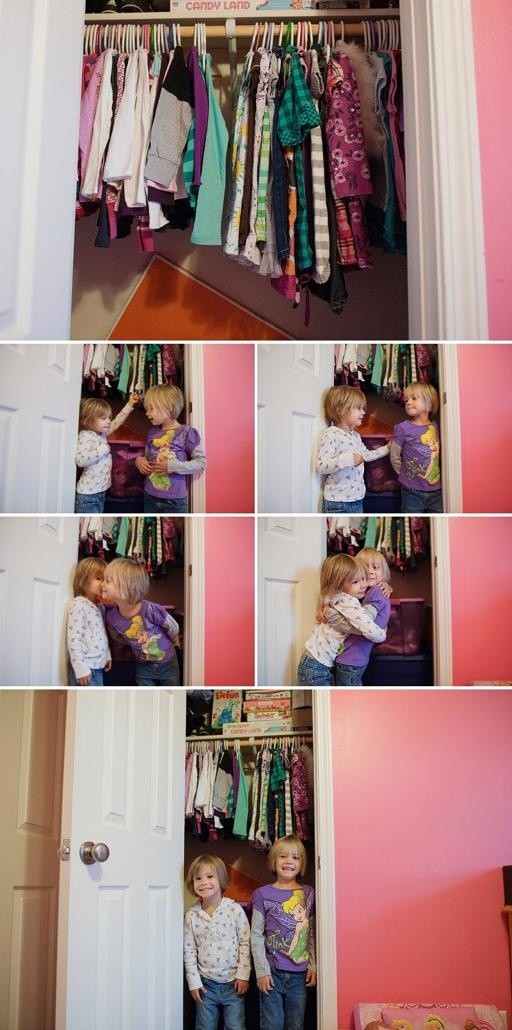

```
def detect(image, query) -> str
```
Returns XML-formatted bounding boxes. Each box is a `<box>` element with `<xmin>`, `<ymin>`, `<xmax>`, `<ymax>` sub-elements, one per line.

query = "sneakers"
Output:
<box><xmin>190</xmin><ymin>725</ymin><xmax>210</xmax><ymax>735</ymax></box>
<box><xmin>104</xmin><ymin>0</ymin><xmax>145</xmax><ymax>11</ymax></box>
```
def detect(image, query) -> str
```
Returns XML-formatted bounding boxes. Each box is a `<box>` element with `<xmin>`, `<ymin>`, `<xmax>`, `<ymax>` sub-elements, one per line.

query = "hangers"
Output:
<box><xmin>84</xmin><ymin>19</ymin><xmax>403</xmax><ymax>91</ymax></box>
<box><xmin>186</xmin><ymin>737</ymin><xmax>311</xmax><ymax>757</ymax></box>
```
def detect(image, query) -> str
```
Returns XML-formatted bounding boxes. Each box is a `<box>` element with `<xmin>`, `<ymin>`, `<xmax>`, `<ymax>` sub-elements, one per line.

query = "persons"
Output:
<box><xmin>297</xmin><ymin>553</ymin><xmax>392</xmax><ymax>686</ymax></box>
<box><xmin>250</xmin><ymin>833</ymin><xmax>317</xmax><ymax>1029</ymax></box>
<box><xmin>315</xmin><ymin>385</ymin><xmax>392</xmax><ymax>513</ymax></box>
<box><xmin>391</xmin><ymin>380</ymin><xmax>444</xmax><ymax>513</ymax></box>
<box><xmin>101</xmin><ymin>558</ymin><xmax>182</xmax><ymax>686</ymax></box>
<box><xmin>183</xmin><ymin>853</ymin><xmax>252</xmax><ymax>1030</ymax></box>
<box><xmin>134</xmin><ymin>383</ymin><xmax>206</xmax><ymax>513</ymax></box>
<box><xmin>67</xmin><ymin>557</ymin><xmax>113</xmax><ymax>686</ymax></box>
<box><xmin>316</xmin><ymin>548</ymin><xmax>391</xmax><ymax>686</ymax></box>
<box><xmin>75</xmin><ymin>391</ymin><xmax>139</xmax><ymax>513</ymax></box>
